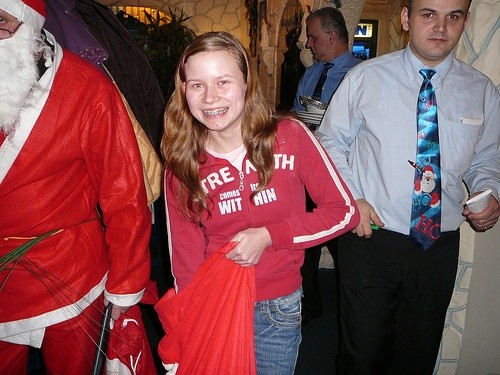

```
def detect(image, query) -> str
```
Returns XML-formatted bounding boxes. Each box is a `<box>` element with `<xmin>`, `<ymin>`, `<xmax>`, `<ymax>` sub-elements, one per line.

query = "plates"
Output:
<box><xmin>296</xmin><ymin>110</ymin><xmax>325</xmax><ymax>125</ymax></box>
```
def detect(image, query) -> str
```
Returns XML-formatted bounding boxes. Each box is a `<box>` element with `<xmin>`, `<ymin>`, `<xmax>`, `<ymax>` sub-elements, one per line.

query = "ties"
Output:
<box><xmin>409</xmin><ymin>68</ymin><xmax>442</xmax><ymax>253</ymax></box>
<box><xmin>311</xmin><ymin>63</ymin><xmax>335</xmax><ymax>100</ymax></box>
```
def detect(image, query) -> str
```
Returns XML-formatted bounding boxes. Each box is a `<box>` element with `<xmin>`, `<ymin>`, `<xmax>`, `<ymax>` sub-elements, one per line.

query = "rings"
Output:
<box><xmin>484</xmin><ymin>226</ymin><xmax>488</xmax><ymax>230</ymax></box>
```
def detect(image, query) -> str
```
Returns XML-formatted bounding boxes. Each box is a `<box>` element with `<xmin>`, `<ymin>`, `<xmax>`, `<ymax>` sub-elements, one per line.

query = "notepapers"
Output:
<box><xmin>465</xmin><ymin>187</ymin><xmax>494</xmax><ymax>214</ymax></box>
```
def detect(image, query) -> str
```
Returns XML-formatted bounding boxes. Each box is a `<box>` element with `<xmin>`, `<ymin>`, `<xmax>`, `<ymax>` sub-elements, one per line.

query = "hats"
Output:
<box><xmin>0</xmin><ymin>0</ymin><xmax>47</xmax><ymax>34</ymax></box>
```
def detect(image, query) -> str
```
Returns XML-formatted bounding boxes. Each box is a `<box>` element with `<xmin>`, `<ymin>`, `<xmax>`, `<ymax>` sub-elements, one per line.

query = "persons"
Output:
<box><xmin>312</xmin><ymin>0</ymin><xmax>499</xmax><ymax>375</ymax></box>
<box><xmin>159</xmin><ymin>30</ymin><xmax>360</xmax><ymax>375</ymax></box>
<box><xmin>288</xmin><ymin>6</ymin><xmax>365</xmax><ymax>329</ymax></box>
<box><xmin>0</xmin><ymin>0</ymin><xmax>151</xmax><ymax>375</ymax></box>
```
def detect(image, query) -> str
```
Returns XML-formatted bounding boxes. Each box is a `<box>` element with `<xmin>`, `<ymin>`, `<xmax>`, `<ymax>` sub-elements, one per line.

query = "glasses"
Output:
<box><xmin>0</xmin><ymin>22</ymin><xmax>23</xmax><ymax>40</ymax></box>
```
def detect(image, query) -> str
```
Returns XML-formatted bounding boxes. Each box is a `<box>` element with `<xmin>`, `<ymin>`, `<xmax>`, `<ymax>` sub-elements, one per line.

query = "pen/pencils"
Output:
<box><xmin>408</xmin><ymin>160</ymin><xmax>425</xmax><ymax>173</ymax></box>
<box><xmin>371</xmin><ymin>224</ymin><xmax>380</xmax><ymax>230</ymax></box>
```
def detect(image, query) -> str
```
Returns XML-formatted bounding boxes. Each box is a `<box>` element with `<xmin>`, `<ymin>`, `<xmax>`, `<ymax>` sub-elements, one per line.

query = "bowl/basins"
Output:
<box><xmin>299</xmin><ymin>95</ymin><xmax>327</xmax><ymax>110</ymax></box>
